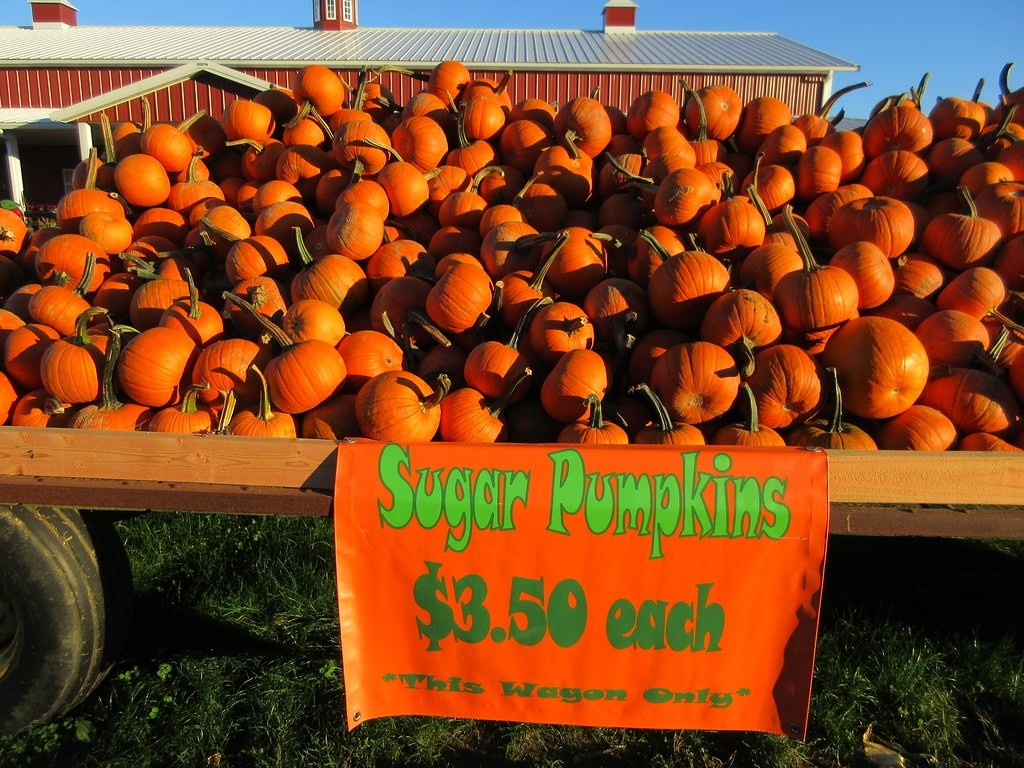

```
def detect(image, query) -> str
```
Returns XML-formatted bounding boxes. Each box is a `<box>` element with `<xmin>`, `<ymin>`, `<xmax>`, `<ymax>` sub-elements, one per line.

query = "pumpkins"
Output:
<box><xmin>0</xmin><ymin>61</ymin><xmax>1024</xmax><ymax>451</ymax></box>
